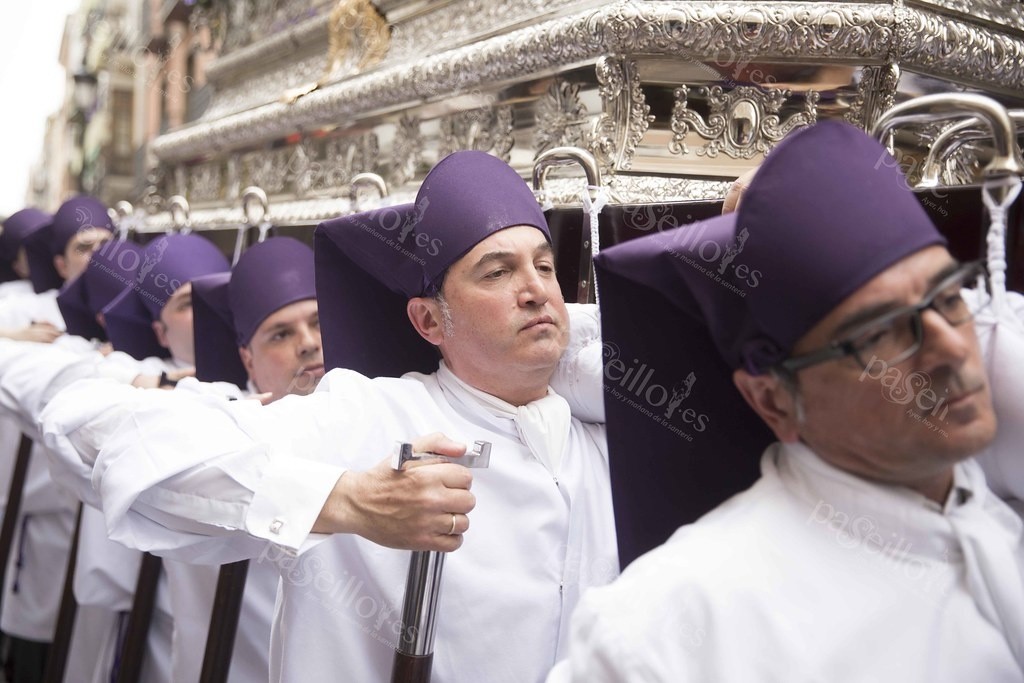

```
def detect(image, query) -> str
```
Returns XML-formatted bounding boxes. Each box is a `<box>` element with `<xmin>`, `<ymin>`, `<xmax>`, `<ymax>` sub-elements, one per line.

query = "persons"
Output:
<box><xmin>103</xmin><ymin>148</ymin><xmax>760</xmax><ymax>681</ymax></box>
<box><xmin>546</xmin><ymin>115</ymin><xmax>1024</xmax><ymax>683</ymax></box>
<box><xmin>0</xmin><ymin>194</ymin><xmax>325</xmax><ymax>683</ymax></box>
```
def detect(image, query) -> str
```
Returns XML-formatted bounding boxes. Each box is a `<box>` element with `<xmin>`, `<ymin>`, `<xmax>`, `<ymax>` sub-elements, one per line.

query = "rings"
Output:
<box><xmin>448</xmin><ymin>512</ymin><xmax>458</xmax><ymax>537</ymax></box>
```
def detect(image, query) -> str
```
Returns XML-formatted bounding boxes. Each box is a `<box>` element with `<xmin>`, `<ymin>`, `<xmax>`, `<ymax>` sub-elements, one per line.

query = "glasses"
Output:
<box><xmin>779</xmin><ymin>258</ymin><xmax>992</xmax><ymax>377</ymax></box>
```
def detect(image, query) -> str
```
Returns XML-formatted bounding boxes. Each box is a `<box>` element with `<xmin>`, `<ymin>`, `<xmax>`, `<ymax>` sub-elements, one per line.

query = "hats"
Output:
<box><xmin>1</xmin><ymin>150</ymin><xmax>556</xmax><ymax>392</ymax></box>
<box><xmin>591</xmin><ymin>116</ymin><xmax>950</xmax><ymax>576</ymax></box>
